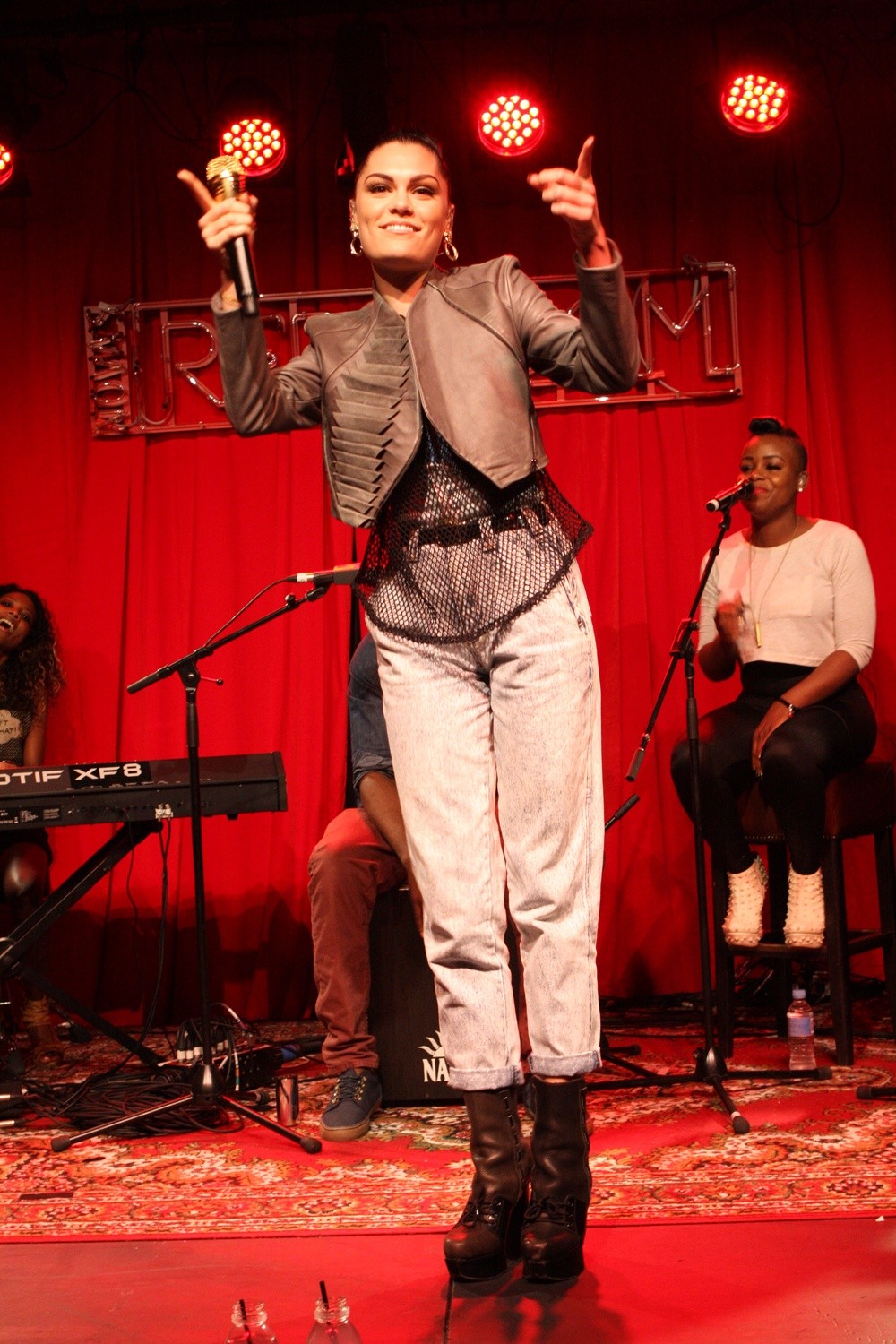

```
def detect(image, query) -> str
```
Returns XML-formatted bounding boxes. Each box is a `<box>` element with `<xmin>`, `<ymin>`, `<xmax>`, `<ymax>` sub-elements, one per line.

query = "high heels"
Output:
<box><xmin>783</xmin><ymin>863</ymin><xmax>826</xmax><ymax>948</ymax></box>
<box><xmin>721</xmin><ymin>853</ymin><xmax>769</xmax><ymax>947</ymax></box>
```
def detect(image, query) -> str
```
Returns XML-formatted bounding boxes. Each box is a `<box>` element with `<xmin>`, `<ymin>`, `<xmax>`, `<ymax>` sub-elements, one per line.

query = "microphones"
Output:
<box><xmin>204</xmin><ymin>155</ymin><xmax>262</xmax><ymax>318</ymax></box>
<box><xmin>286</xmin><ymin>559</ymin><xmax>386</xmax><ymax>588</ymax></box>
<box><xmin>705</xmin><ymin>477</ymin><xmax>755</xmax><ymax>513</ymax></box>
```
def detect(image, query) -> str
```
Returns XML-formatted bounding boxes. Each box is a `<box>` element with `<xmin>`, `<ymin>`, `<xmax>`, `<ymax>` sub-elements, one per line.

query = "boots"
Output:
<box><xmin>445</xmin><ymin>1089</ymin><xmax>529</xmax><ymax>1285</ymax></box>
<box><xmin>521</xmin><ymin>1074</ymin><xmax>593</xmax><ymax>1283</ymax></box>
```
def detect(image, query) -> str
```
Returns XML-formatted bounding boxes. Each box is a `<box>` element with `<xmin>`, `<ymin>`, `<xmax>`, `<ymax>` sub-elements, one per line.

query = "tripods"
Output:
<box><xmin>589</xmin><ymin>518</ymin><xmax>834</xmax><ymax>1138</ymax></box>
<box><xmin>50</xmin><ymin>587</ymin><xmax>326</xmax><ymax>1157</ymax></box>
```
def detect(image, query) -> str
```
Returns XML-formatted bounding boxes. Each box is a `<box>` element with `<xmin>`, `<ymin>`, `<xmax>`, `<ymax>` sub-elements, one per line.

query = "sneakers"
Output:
<box><xmin>319</xmin><ymin>1067</ymin><xmax>384</xmax><ymax>1140</ymax></box>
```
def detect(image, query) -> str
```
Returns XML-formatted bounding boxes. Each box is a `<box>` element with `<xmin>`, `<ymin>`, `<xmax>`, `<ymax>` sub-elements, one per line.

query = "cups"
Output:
<box><xmin>276</xmin><ymin>1075</ymin><xmax>299</xmax><ymax>1126</ymax></box>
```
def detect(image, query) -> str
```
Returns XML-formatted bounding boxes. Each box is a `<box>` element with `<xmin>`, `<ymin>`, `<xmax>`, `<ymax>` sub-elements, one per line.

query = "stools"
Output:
<box><xmin>714</xmin><ymin>760</ymin><xmax>896</xmax><ymax>1067</ymax></box>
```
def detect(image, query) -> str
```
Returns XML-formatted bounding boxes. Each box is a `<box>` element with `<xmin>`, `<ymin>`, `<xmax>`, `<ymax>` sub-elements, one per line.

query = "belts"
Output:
<box><xmin>402</xmin><ymin>509</ymin><xmax>549</xmax><ymax>547</ymax></box>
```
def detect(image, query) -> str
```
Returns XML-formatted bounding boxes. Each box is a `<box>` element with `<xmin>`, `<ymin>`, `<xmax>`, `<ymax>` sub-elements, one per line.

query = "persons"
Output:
<box><xmin>668</xmin><ymin>417</ymin><xmax>877</xmax><ymax>949</ymax></box>
<box><xmin>307</xmin><ymin>640</ymin><xmax>412</xmax><ymax>1140</ymax></box>
<box><xmin>169</xmin><ymin>126</ymin><xmax>640</xmax><ymax>1294</ymax></box>
<box><xmin>0</xmin><ymin>579</ymin><xmax>66</xmax><ymax>971</ymax></box>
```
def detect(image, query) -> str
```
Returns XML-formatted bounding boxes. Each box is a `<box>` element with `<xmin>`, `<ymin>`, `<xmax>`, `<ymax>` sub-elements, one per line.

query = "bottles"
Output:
<box><xmin>226</xmin><ymin>1298</ymin><xmax>278</xmax><ymax>1344</ymax></box>
<box><xmin>306</xmin><ymin>1298</ymin><xmax>363</xmax><ymax>1344</ymax></box>
<box><xmin>785</xmin><ymin>989</ymin><xmax>818</xmax><ymax>1070</ymax></box>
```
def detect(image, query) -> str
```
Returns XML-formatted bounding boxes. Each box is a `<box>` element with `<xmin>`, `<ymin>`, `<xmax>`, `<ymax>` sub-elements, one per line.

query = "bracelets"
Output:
<box><xmin>775</xmin><ymin>695</ymin><xmax>801</xmax><ymax>716</ymax></box>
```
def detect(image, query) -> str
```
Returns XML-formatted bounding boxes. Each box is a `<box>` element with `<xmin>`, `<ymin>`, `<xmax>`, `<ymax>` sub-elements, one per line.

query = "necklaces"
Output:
<box><xmin>748</xmin><ymin>510</ymin><xmax>802</xmax><ymax>650</ymax></box>
